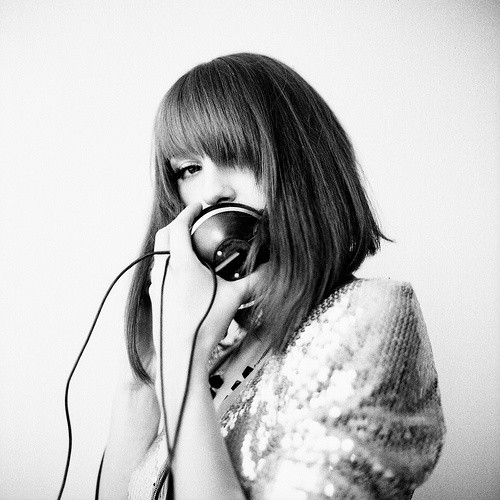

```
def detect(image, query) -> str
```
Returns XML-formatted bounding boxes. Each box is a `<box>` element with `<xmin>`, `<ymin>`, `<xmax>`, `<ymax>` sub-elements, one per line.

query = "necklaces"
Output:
<box><xmin>150</xmin><ymin>314</ymin><xmax>274</xmax><ymax>500</ymax></box>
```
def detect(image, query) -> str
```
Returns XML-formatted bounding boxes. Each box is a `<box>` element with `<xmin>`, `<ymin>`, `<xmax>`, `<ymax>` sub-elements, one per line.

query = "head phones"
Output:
<box><xmin>187</xmin><ymin>203</ymin><xmax>267</xmax><ymax>310</ymax></box>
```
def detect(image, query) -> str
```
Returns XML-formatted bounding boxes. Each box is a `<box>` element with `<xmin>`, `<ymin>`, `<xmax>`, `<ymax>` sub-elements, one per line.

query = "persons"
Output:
<box><xmin>94</xmin><ymin>52</ymin><xmax>448</xmax><ymax>500</ymax></box>
